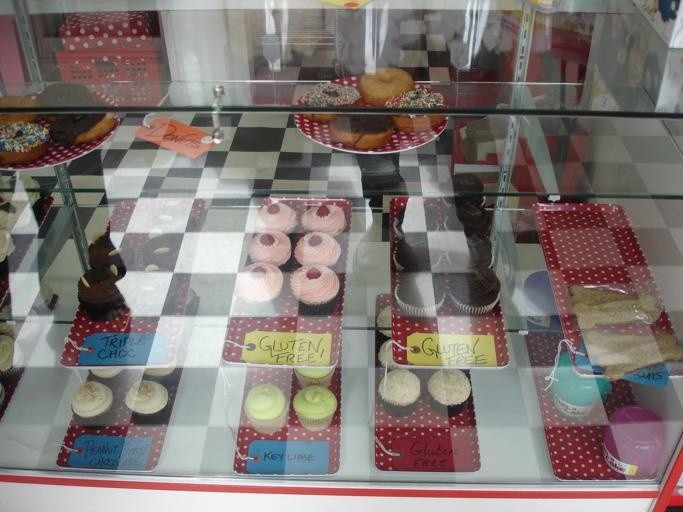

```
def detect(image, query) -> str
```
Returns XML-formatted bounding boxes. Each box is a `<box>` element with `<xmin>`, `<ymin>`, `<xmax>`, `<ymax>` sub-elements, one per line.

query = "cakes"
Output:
<box><xmin>0</xmin><ymin>173</ymin><xmax>54</xmax><ymax>409</ymax></box>
<box><xmin>1</xmin><ymin>174</ymin><xmax>50</xmax><ymax>401</ymax></box>
<box><xmin>69</xmin><ymin>170</ymin><xmax>502</xmax><ymax>434</ymax></box>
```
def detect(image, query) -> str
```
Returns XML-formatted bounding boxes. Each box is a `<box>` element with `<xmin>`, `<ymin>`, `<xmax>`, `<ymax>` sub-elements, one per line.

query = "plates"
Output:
<box><xmin>58</xmin><ymin>197</ymin><xmax>205</xmax><ymax>369</ymax></box>
<box><xmin>373</xmin><ymin>291</ymin><xmax>482</xmax><ymax>474</ymax></box>
<box><xmin>0</xmin><ymin>288</ymin><xmax>202</xmax><ymax>475</ymax></box>
<box><xmin>292</xmin><ymin>74</ymin><xmax>450</xmax><ymax>157</ymax></box>
<box><xmin>231</xmin><ymin>332</ymin><xmax>343</xmax><ymax>477</ymax></box>
<box><xmin>388</xmin><ymin>195</ymin><xmax>510</xmax><ymax>370</ymax></box>
<box><xmin>0</xmin><ymin>89</ymin><xmax>120</xmax><ymax>171</ymax></box>
<box><xmin>531</xmin><ymin>202</ymin><xmax>683</xmax><ymax>378</ymax></box>
<box><xmin>524</xmin><ymin>331</ymin><xmax>660</xmax><ymax>483</ymax></box>
<box><xmin>221</xmin><ymin>196</ymin><xmax>351</xmax><ymax>369</ymax></box>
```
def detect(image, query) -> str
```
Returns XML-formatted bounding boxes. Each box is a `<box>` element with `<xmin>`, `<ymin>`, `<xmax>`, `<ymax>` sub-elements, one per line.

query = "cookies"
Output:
<box><xmin>570</xmin><ymin>285</ymin><xmax>682</xmax><ymax>382</ymax></box>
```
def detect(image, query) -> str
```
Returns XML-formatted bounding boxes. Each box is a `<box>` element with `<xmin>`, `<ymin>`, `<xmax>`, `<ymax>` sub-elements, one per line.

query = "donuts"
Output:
<box><xmin>1</xmin><ymin>95</ymin><xmax>40</xmax><ymax>123</ymax></box>
<box><xmin>357</xmin><ymin>66</ymin><xmax>416</xmax><ymax>108</ymax></box>
<box><xmin>328</xmin><ymin>103</ymin><xmax>396</xmax><ymax>148</ymax></box>
<box><xmin>304</xmin><ymin>83</ymin><xmax>363</xmax><ymax>126</ymax></box>
<box><xmin>49</xmin><ymin>97</ymin><xmax>114</xmax><ymax>145</ymax></box>
<box><xmin>0</xmin><ymin>83</ymin><xmax>114</xmax><ymax>167</ymax></box>
<box><xmin>42</xmin><ymin>83</ymin><xmax>92</xmax><ymax>124</ymax></box>
<box><xmin>0</xmin><ymin>120</ymin><xmax>50</xmax><ymax>165</ymax></box>
<box><xmin>382</xmin><ymin>86</ymin><xmax>445</xmax><ymax>134</ymax></box>
<box><xmin>304</xmin><ymin>64</ymin><xmax>447</xmax><ymax>149</ymax></box>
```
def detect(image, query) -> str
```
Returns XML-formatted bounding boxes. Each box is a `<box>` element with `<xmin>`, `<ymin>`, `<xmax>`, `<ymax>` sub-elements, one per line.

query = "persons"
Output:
<box><xmin>322</xmin><ymin>3</ymin><xmax>521</xmax><ymax>301</ymax></box>
<box><xmin>0</xmin><ymin>0</ymin><xmax>127</xmax><ymax>370</ymax></box>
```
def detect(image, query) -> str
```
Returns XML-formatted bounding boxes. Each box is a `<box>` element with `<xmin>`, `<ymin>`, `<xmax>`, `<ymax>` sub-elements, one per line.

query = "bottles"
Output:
<box><xmin>550</xmin><ymin>351</ymin><xmax>614</xmax><ymax>422</ymax></box>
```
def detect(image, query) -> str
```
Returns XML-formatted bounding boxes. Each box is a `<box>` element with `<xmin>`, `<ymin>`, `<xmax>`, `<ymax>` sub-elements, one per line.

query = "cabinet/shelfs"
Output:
<box><xmin>0</xmin><ymin>0</ymin><xmax>683</xmax><ymax>512</ymax></box>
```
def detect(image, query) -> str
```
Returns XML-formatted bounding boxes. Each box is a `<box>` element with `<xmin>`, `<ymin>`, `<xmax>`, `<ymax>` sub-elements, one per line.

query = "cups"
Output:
<box><xmin>601</xmin><ymin>404</ymin><xmax>667</xmax><ymax>481</ymax></box>
